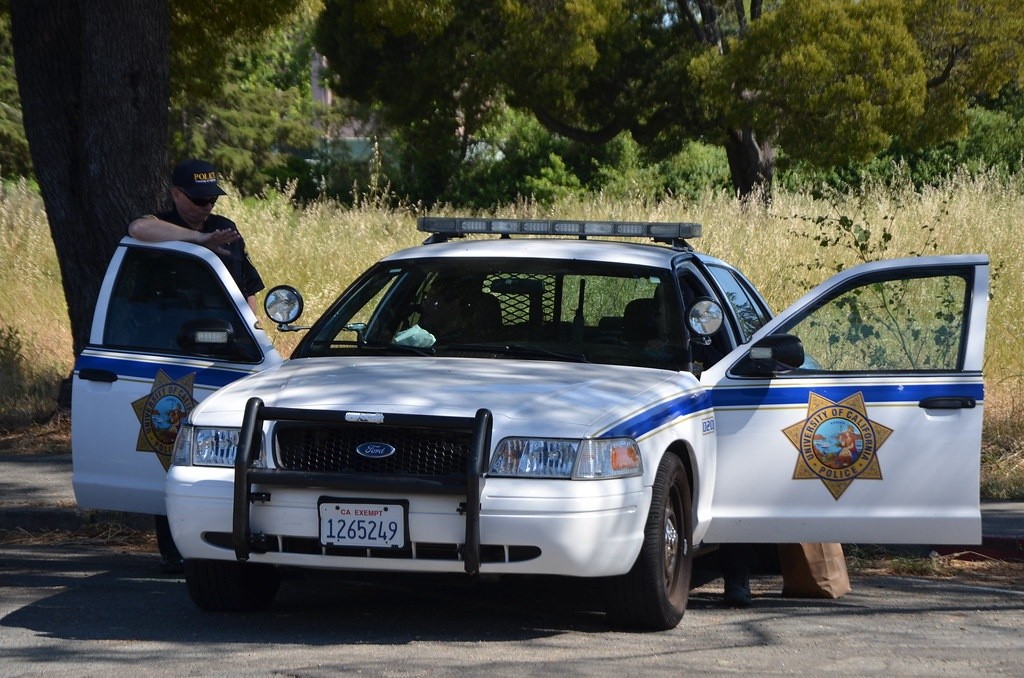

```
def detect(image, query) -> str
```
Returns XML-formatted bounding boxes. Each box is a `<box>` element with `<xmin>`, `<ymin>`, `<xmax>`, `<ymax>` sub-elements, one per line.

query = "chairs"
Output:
<box><xmin>621</xmin><ymin>298</ymin><xmax>657</xmax><ymax>343</ymax></box>
<box><xmin>418</xmin><ymin>273</ymin><xmax>502</xmax><ymax>346</ymax></box>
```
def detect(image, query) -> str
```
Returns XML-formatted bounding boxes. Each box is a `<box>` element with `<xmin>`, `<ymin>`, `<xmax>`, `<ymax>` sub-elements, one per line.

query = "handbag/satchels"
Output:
<box><xmin>776</xmin><ymin>542</ymin><xmax>853</xmax><ymax>600</ymax></box>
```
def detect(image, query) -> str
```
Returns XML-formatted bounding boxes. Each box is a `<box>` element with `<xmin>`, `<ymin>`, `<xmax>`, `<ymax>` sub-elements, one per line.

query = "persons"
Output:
<box><xmin>624</xmin><ymin>281</ymin><xmax>753</xmax><ymax>607</ymax></box>
<box><xmin>127</xmin><ymin>159</ymin><xmax>264</xmax><ymax>568</ymax></box>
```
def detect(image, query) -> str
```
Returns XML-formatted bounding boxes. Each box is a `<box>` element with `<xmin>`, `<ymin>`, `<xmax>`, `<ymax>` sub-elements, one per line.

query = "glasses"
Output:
<box><xmin>177</xmin><ymin>187</ymin><xmax>219</xmax><ymax>207</ymax></box>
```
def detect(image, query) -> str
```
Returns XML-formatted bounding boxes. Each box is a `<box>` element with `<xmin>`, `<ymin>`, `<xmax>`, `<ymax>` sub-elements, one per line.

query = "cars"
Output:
<box><xmin>68</xmin><ymin>214</ymin><xmax>992</xmax><ymax>632</ymax></box>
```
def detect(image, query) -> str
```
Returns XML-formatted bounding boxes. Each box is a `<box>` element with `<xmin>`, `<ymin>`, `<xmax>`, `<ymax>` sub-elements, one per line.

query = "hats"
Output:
<box><xmin>173</xmin><ymin>160</ymin><xmax>228</xmax><ymax>198</ymax></box>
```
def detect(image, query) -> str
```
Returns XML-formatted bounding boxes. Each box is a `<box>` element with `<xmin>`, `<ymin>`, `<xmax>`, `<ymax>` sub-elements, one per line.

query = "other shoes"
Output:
<box><xmin>163</xmin><ymin>553</ymin><xmax>183</xmax><ymax>574</ymax></box>
<box><xmin>724</xmin><ymin>582</ymin><xmax>754</xmax><ymax>607</ymax></box>
